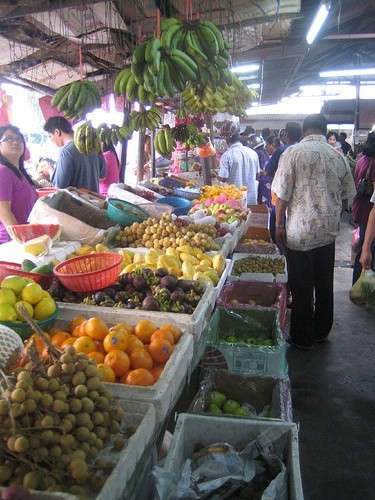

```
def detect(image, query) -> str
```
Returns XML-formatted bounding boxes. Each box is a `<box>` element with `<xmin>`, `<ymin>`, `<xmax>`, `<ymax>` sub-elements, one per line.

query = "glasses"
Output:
<box><xmin>0</xmin><ymin>136</ymin><xmax>23</xmax><ymax>143</ymax></box>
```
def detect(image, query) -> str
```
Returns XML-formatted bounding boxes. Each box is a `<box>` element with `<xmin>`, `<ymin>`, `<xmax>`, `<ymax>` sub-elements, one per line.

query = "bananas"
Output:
<box><xmin>171</xmin><ymin>123</ymin><xmax>198</xmax><ymax>148</ymax></box>
<box><xmin>110</xmin><ymin>123</ymin><xmax>131</xmax><ymax>145</ymax></box>
<box><xmin>184</xmin><ymin>131</ymin><xmax>210</xmax><ymax>150</ymax></box>
<box><xmin>114</xmin><ymin>18</ymin><xmax>260</xmax><ymax>118</ymax></box>
<box><xmin>154</xmin><ymin>124</ymin><xmax>176</xmax><ymax>159</ymax></box>
<box><xmin>51</xmin><ymin>81</ymin><xmax>103</xmax><ymax>122</ymax></box>
<box><xmin>129</xmin><ymin>107</ymin><xmax>167</xmax><ymax>132</ymax></box>
<box><xmin>74</xmin><ymin>123</ymin><xmax>99</xmax><ymax>154</ymax></box>
<box><xmin>99</xmin><ymin>122</ymin><xmax>111</xmax><ymax>145</ymax></box>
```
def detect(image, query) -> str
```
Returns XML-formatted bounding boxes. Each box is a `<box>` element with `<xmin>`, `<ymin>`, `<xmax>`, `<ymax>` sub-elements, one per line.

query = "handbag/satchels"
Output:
<box><xmin>356</xmin><ymin>179</ymin><xmax>372</xmax><ymax>204</ymax></box>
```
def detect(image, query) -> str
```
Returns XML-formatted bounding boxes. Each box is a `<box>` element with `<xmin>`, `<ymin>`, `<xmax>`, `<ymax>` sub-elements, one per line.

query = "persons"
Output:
<box><xmin>0</xmin><ymin>125</ymin><xmax>38</xmax><ymax>243</ymax></box>
<box><xmin>359</xmin><ymin>190</ymin><xmax>375</xmax><ymax>269</ymax></box>
<box><xmin>243</xmin><ymin>121</ymin><xmax>285</xmax><ymax>170</ymax></box>
<box><xmin>352</xmin><ymin>129</ymin><xmax>375</xmax><ymax>282</ymax></box>
<box><xmin>132</xmin><ymin>134</ymin><xmax>150</xmax><ymax>181</ymax></box>
<box><xmin>259</xmin><ymin>123</ymin><xmax>300</xmax><ymax>256</ymax></box>
<box><xmin>38</xmin><ymin>116</ymin><xmax>106</xmax><ymax>194</ymax></box>
<box><xmin>100</xmin><ymin>140</ymin><xmax>120</xmax><ymax>197</ymax></box>
<box><xmin>327</xmin><ymin>132</ymin><xmax>341</xmax><ymax>153</ymax></box>
<box><xmin>271</xmin><ymin>114</ymin><xmax>358</xmax><ymax>350</ymax></box>
<box><xmin>214</xmin><ymin>132</ymin><xmax>259</xmax><ymax>205</ymax></box>
<box><xmin>339</xmin><ymin>133</ymin><xmax>351</xmax><ymax>154</ymax></box>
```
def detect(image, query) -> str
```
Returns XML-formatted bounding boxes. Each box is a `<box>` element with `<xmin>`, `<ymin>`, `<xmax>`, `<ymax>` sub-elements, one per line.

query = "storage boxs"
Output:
<box><xmin>0</xmin><ymin>398</ymin><xmax>155</xmax><ymax>500</ymax></box>
<box><xmin>55</xmin><ymin>282</ymin><xmax>215</xmax><ymax>373</ymax></box>
<box><xmin>247</xmin><ymin>204</ymin><xmax>270</xmax><ymax>228</ymax></box>
<box><xmin>229</xmin><ymin>9</ymin><xmax>260</xmax><ymax>73</ymax></box>
<box><xmin>244</xmin><ymin>228</ymin><xmax>272</xmax><ymax>236</ymax></box>
<box><xmin>238</xmin><ymin>236</ymin><xmax>273</xmax><ymax>244</ymax></box>
<box><xmin>55</xmin><ymin>318</ymin><xmax>194</xmax><ymax>430</ymax></box>
<box><xmin>185</xmin><ymin>376</ymin><xmax>289</xmax><ymax>422</ymax></box>
<box><xmin>154</xmin><ymin>414</ymin><xmax>304</xmax><ymax>500</ymax></box>
<box><xmin>217</xmin><ymin>281</ymin><xmax>286</xmax><ymax>330</ymax></box>
<box><xmin>306</xmin><ymin>0</ymin><xmax>331</xmax><ymax>44</ymax></box>
<box><xmin>114</xmin><ymin>246</ymin><xmax>233</xmax><ymax>298</ymax></box>
<box><xmin>27</xmin><ymin>190</ymin><xmax>121</xmax><ymax>248</ymax></box>
<box><xmin>201</xmin><ymin>307</ymin><xmax>285</xmax><ymax>376</ymax></box>
<box><xmin>233</xmin><ymin>244</ymin><xmax>280</xmax><ymax>254</ymax></box>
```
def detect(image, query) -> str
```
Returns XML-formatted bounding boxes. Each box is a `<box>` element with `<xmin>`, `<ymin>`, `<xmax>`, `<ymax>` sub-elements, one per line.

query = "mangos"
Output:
<box><xmin>24</xmin><ymin>236</ymin><xmax>52</xmax><ymax>255</ymax></box>
<box><xmin>70</xmin><ymin>244</ymin><xmax>223</xmax><ymax>286</ymax></box>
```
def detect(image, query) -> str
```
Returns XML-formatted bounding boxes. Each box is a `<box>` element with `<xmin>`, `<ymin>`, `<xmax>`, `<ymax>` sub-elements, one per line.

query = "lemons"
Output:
<box><xmin>0</xmin><ymin>275</ymin><xmax>55</xmax><ymax>323</ymax></box>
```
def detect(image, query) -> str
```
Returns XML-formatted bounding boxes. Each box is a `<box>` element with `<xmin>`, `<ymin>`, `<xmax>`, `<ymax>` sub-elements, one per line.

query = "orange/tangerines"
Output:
<box><xmin>18</xmin><ymin>314</ymin><xmax>180</xmax><ymax>385</ymax></box>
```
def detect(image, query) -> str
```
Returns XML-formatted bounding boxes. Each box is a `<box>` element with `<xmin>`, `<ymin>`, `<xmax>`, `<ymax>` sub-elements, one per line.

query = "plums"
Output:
<box><xmin>176</xmin><ymin>217</ymin><xmax>228</xmax><ymax>237</ymax></box>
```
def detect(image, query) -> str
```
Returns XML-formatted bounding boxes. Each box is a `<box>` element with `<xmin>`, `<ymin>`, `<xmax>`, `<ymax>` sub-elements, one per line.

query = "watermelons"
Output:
<box><xmin>7</xmin><ymin>224</ymin><xmax>63</xmax><ymax>245</ymax></box>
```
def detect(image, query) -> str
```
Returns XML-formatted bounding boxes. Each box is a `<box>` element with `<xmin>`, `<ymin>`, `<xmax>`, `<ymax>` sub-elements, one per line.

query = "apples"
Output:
<box><xmin>230</xmin><ymin>297</ymin><xmax>238</xmax><ymax>303</ymax></box>
<box><xmin>206</xmin><ymin>387</ymin><xmax>249</xmax><ymax>415</ymax></box>
<box><xmin>216</xmin><ymin>335</ymin><xmax>273</xmax><ymax>346</ymax></box>
<box><xmin>187</xmin><ymin>202</ymin><xmax>248</xmax><ymax>223</ymax></box>
<box><xmin>249</xmin><ymin>299</ymin><xmax>256</xmax><ymax>305</ymax></box>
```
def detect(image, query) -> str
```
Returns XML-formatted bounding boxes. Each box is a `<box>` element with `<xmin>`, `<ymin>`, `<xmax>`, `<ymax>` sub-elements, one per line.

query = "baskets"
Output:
<box><xmin>0</xmin><ymin>301</ymin><xmax>59</xmax><ymax>344</ymax></box>
<box><xmin>174</xmin><ymin>187</ymin><xmax>201</xmax><ymax>199</ymax></box>
<box><xmin>1</xmin><ymin>261</ymin><xmax>55</xmax><ymax>291</ymax></box>
<box><xmin>52</xmin><ymin>253</ymin><xmax>124</xmax><ymax>293</ymax></box>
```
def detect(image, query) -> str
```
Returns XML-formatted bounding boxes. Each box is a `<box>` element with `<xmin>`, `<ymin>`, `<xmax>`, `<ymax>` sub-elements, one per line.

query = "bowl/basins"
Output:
<box><xmin>154</xmin><ymin>196</ymin><xmax>192</xmax><ymax>217</ymax></box>
<box><xmin>107</xmin><ymin>198</ymin><xmax>150</xmax><ymax>227</ymax></box>
<box><xmin>136</xmin><ymin>203</ymin><xmax>175</xmax><ymax>220</ymax></box>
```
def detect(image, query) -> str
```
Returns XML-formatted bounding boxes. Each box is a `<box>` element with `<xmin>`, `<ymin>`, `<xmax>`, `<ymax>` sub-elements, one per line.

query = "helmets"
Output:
<box><xmin>247</xmin><ymin>135</ymin><xmax>265</xmax><ymax>149</ymax></box>
